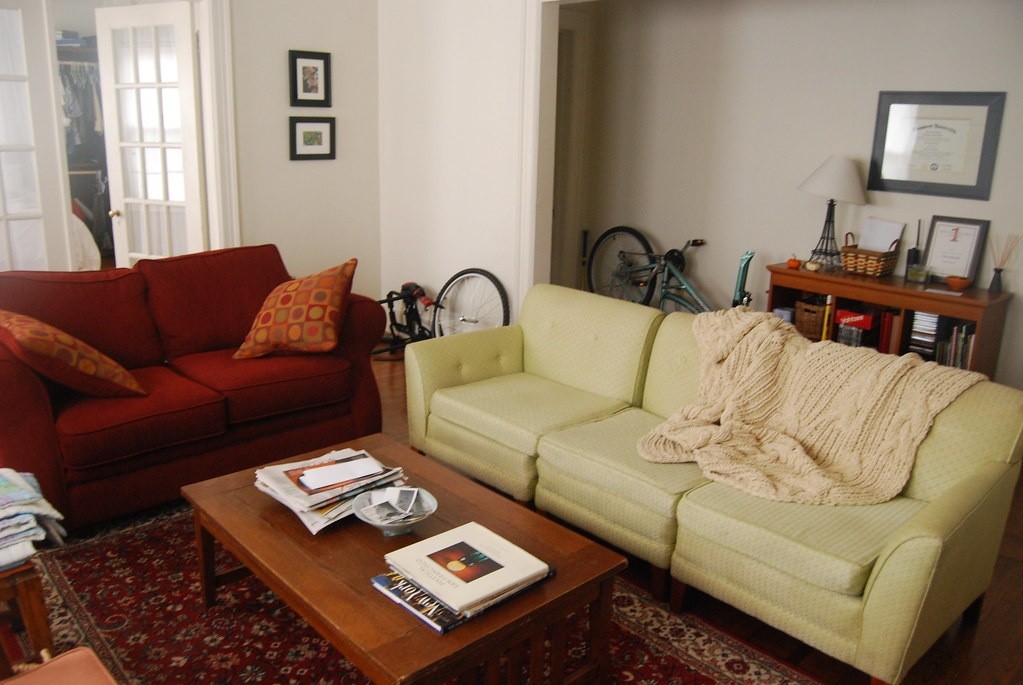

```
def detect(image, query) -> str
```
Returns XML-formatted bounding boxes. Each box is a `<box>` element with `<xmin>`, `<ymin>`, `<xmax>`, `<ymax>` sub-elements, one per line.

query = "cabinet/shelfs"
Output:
<box><xmin>766</xmin><ymin>262</ymin><xmax>1015</xmax><ymax>383</ymax></box>
<box><xmin>58</xmin><ymin>44</ymin><xmax>115</xmax><ymax>265</ymax></box>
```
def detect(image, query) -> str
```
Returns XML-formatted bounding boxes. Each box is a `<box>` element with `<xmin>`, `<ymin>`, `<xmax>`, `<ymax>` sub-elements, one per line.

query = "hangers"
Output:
<box><xmin>58</xmin><ymin>60</ymin><xmax>97</xmax><ymax>85</ymax></box>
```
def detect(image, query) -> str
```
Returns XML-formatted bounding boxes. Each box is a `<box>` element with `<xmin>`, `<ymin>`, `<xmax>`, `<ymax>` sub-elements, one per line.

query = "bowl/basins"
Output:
<box><xmin>946</xmin><ymin>276</ymin><xmax>972</xmax><ymax>292</ymax></box>
<box><xmin>353</xmin><ymin>487</ymin><xmax>438</xmax><ymax>536</ymax></box>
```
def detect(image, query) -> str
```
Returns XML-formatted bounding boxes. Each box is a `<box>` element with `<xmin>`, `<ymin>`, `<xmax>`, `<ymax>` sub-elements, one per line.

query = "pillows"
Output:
<box><xmin>232</xmin><ymin>258</ymin><xmax>358</xmax><ymax>359</ymax></box>
<box><xmin>0</xmin><ymin>311</ymin><xmax>148</xmax><ymax>400</ymax></box>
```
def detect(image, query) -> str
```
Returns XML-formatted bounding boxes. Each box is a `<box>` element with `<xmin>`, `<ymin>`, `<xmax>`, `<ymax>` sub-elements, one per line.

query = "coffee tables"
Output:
<box><xmin>179</xmin><ymin>432</ymin><xmax>629</xmax><ymax>684</ymax></box>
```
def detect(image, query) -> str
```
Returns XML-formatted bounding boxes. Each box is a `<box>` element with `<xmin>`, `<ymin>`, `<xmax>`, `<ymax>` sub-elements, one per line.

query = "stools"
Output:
<box><xmin>0</xmin><ymin>562</ymin><xmax>55</xmax><ymax>677</ymax></box>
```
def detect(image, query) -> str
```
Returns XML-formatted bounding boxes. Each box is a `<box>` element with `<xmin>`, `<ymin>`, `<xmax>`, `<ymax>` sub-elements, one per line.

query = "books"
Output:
<box><xmin>255</xmin><ymin>447</ymin><xmax>409</xmax><ymax>535</ymax></box>
<box><xmin>936</xmin><ymin>322</ymin><xmax>976</xmax><ymax>371</ymax></box>
<box><xmin>369</xmin><ymin>521</ymin><xmax>558</xmax><ymax>635</ymax></box>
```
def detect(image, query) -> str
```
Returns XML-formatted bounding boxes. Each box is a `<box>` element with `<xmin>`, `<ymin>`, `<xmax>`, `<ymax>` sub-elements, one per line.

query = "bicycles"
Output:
<box><xmin>373</xmin><ymin>268</ymin><xmax>510</xmax><ymax>357</ymax></box>
<box><xmin>587</xmin><ymin>225</ymin><xmax>756</xmax><ymax>319</ymax></box>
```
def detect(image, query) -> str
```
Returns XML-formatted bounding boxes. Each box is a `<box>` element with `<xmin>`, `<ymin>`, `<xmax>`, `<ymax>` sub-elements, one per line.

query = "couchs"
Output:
<box><xmin>0</xmin><ymin>243</ymin><xmax>386</xmax><ymax>544</ymax></box>
<box><xmin>404</xmin><ymin>284</ymin><xmax>1023</xmax><ymax>685</ymax></box>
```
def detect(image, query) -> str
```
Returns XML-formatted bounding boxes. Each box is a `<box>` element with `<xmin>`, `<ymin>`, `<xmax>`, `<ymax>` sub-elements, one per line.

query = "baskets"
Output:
<box><xmin>841</xmin><ymin>232</ymin><xmax>901</xmax><ymax>277</ymax></box>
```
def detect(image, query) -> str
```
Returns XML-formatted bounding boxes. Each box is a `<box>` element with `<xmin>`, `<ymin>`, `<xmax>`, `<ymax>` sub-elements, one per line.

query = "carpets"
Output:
<box><xmin>22</xmin><ymin>508</ymin><xmax>828</xmax><ymax>685</ymax></box>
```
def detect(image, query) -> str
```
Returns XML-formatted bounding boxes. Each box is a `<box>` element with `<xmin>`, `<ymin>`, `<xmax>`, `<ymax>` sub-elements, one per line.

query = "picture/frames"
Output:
<box><xmin>289</xmin><ymin>49</ymin><xmax>332</xmax><ymax>108</ymax></box>
<box><xmin>289</xmin><ymin>116</ymin><xmax>335</xmax><ymax>160</ymax></box>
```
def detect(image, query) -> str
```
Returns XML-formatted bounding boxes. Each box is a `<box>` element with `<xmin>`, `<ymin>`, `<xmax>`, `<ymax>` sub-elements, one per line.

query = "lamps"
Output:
<box><xmin>796</xmin><ymin>153</ymin><xmax>865</xmax><ymax>273</ymax></box>
